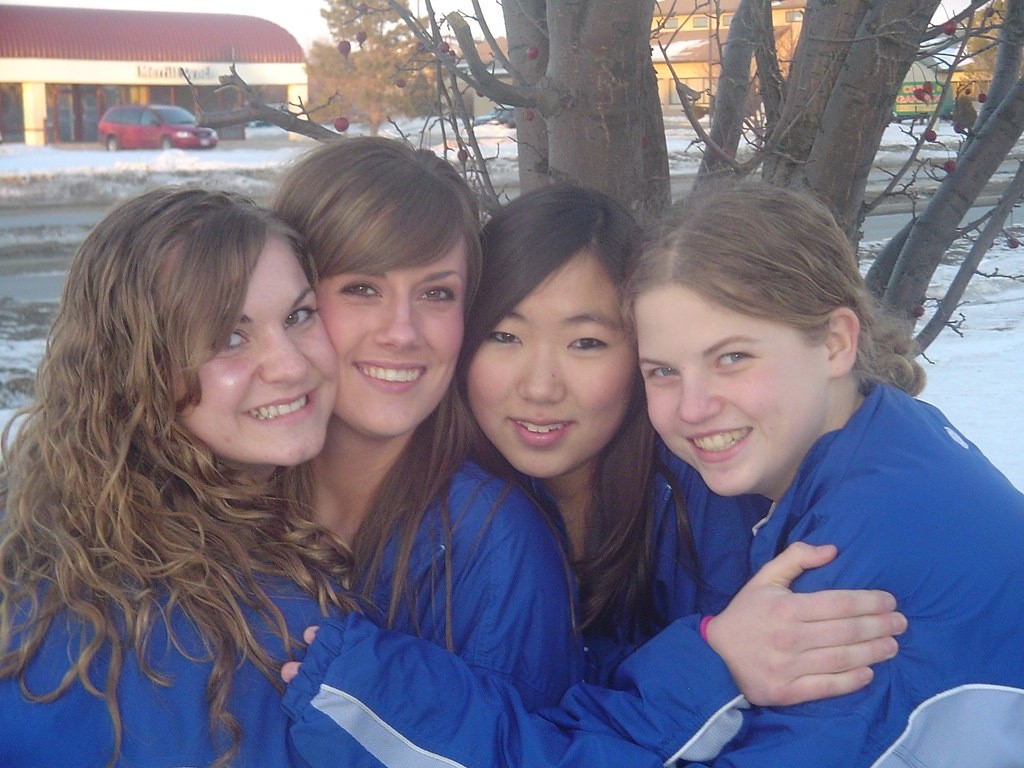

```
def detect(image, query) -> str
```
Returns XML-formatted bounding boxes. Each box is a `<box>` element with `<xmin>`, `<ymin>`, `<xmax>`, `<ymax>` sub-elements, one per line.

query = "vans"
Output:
<box><xmin>97</xmin><ymin>104</ymin><xmax>218</xmax><ymax>152</ymax></box>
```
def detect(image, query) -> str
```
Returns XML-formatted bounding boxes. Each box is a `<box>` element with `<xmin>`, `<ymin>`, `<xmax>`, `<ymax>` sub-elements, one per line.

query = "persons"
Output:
<box><xmin>270</xmin><ymin>136</ymin><xmax>582</xmax><ymax>716</ymax></box>
<box><xmin>460</xmin><ymin>184</ymin><xmax>771</xmax><ymax>677</ymax></box>
<box><xmin>1</xmin><ymin>187</ymin><xmax>907</xmax><ymax>768</ymax></box>
<box><xmin>280</xmin><ymin>181</ymin><xmax>1023</xmax><ymax>768</ymax></box>
